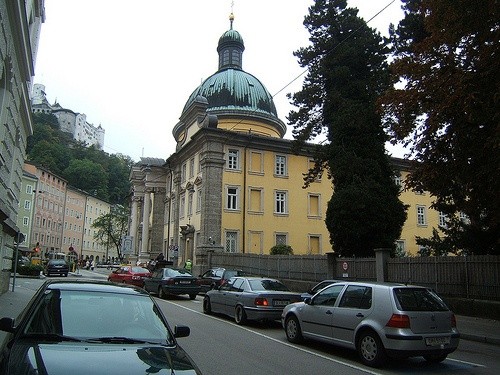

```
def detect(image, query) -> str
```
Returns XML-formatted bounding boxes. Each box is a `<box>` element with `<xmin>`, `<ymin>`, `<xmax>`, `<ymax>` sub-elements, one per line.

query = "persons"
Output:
<box><xmin>184</xmin><ymin>258</ymin><xmax>192</xmax><ymax>272</ymax></box>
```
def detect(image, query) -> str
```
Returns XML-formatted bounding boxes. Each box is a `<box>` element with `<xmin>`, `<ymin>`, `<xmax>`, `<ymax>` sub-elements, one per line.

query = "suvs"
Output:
<box><xmin>199</xmin><ymin>267</ymin><xmax>246</xmax><ymax>293</ymax></box>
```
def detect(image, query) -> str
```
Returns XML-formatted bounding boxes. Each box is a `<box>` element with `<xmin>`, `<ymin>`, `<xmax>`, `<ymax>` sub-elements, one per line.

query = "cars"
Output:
<box><xmin>280</xmin><ymin>281</ymin><xmax>460</xmax><ymax>367</ymax></box>
<box><xmin>0</xmin><ymin>279</ymin><xmax>203</xmax><ymax>375</ymax></box>
<box><xmin>304</xmin><ymin>279</ymin><xmax>343</xmax><ymax>299</ymax></box>
<box><xmin>45</xmin><ymin>259</ymin><xmax>70</xmax><ymax>277</ymax></box>
<box><xmin>143</xmin><ymin>267</ymin><xmax>199</xmax><ymax>299</ymax></box>
<box><xmin>20</xmin><ymin>256</ymin><xmax>30</xmax><ymax>266</ymax></box>
<box><xmin>108</xmin><ymin>266</ymin><xmax>152</xmax><ymax>289</ymax></box>
<box><xmin>202</xmin><ymin>276</ymin><xmax>304</xmax><ymax>326</ymax></box>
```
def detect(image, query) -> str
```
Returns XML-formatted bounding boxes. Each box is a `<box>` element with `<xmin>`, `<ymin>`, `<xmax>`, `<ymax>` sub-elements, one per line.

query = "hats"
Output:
<box><xmin>187</xmin><ymin>259</ymin><xmax>191</xmax><ymax>261</ymax></box>
